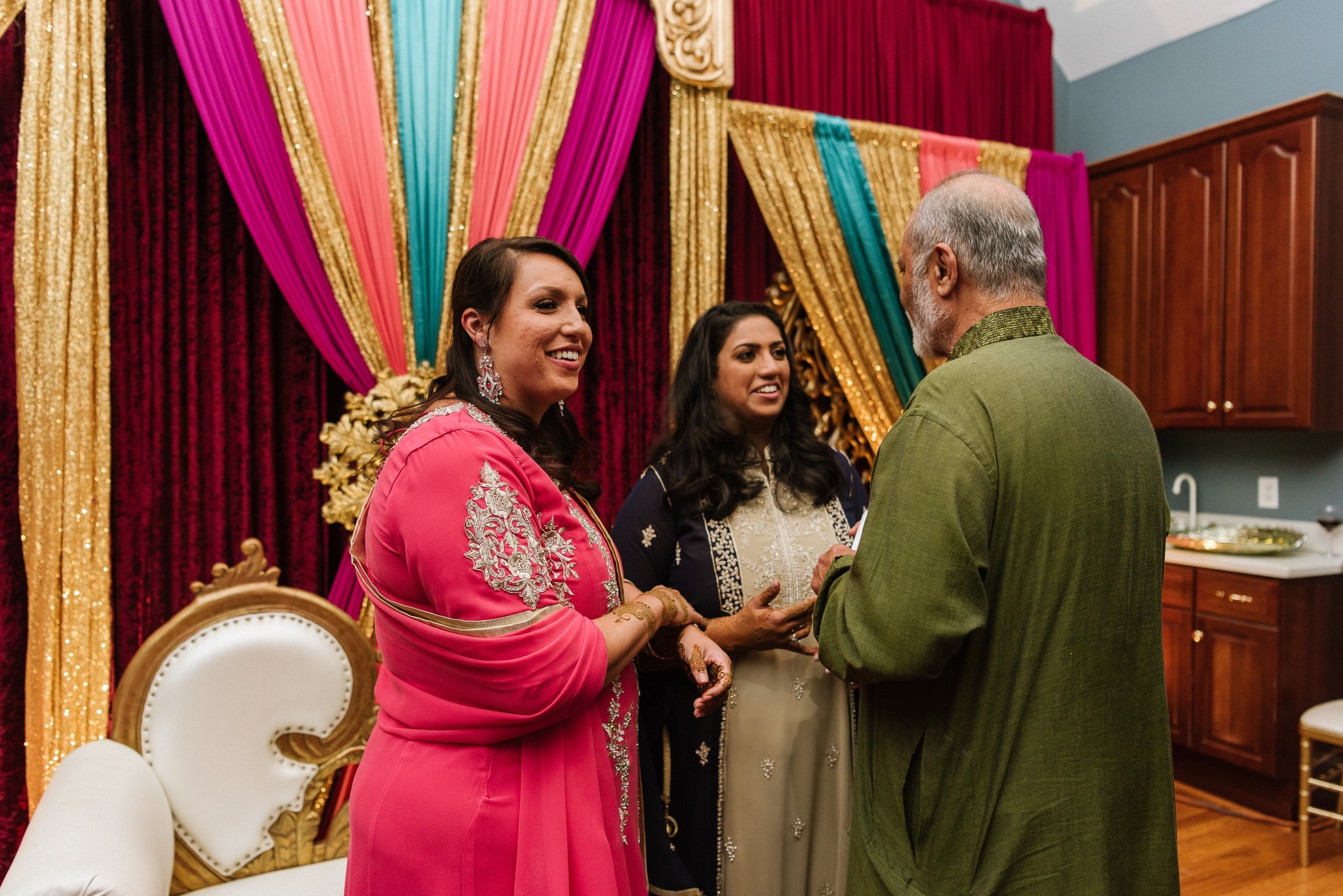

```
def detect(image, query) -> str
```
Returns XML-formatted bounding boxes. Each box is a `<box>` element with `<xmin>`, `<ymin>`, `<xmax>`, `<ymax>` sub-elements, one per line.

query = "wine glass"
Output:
<box><xmin>1317</xmin><ymin>503</ymin><xmax>1343</xmax><ymax>558</ymax></box>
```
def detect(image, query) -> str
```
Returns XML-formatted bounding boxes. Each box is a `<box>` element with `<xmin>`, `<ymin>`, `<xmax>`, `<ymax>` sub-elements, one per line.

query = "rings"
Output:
<box><xmin>792</xmin><ymin>632</ymin><xmax>798</xmax><ymax>642</ymax></box>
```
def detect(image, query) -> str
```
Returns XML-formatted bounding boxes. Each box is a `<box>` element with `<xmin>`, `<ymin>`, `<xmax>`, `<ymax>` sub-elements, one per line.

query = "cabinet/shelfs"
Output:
<box><xmin>1033</xmin><ymin>93</ymin><xmax>1343</xmax><ymax>446</ymax></box>
<box><xmin>1158</xmin><ymin>565</ymin><xmax>1343</xmax><ymax>793</ymax></box>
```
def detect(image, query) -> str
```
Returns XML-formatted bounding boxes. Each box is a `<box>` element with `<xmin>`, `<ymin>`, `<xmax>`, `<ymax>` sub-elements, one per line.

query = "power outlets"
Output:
<box><xmin>1253</xmin><ymin>471</ymin><xmax>1285</xmax><ymax>514</ymax></box>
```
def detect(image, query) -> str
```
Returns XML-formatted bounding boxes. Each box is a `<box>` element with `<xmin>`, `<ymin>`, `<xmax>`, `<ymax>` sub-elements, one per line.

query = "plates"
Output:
<box><xmin>1172</xmin><ymin>525</ymin><xmax>1310</xmax><ymax>555</ymax></box>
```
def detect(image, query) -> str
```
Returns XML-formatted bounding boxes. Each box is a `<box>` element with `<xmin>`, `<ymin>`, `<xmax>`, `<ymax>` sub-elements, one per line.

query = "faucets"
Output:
<box><xmin>1171</xmin><ymin>471</ymin><xmax>1197</xmax><ymax>529</ymax></box>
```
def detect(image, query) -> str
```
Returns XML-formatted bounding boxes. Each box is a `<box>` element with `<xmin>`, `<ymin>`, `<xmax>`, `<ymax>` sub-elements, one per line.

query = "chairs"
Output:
<box><xmin>0</xmin><ymin>532</ymin><xmax>384</xmax><ymax>896</ymax></box>
<box><xmin>1297</xmin><ymin>697</ymin><xmax>1343</xmax><ymax>871</ymax></box>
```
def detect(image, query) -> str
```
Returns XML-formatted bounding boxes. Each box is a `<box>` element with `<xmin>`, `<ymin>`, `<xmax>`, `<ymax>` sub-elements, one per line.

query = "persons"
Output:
<box><xmin>811</xmin><ymin>170</ymin><xmax>1182</xmax><ymax>896</ymax></box>
<box><xmin>609</xmin><ymin>302</ymin><xmax>866</xmax><ymax>896</ymax></box>
<box><xmin>345</xmin><ymin>235</ymin><xmax>732</xmax><ymax>896</ymax></box>
